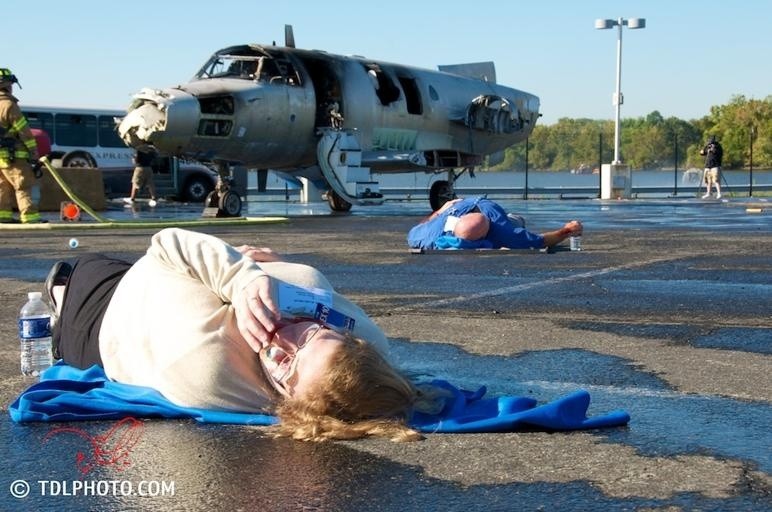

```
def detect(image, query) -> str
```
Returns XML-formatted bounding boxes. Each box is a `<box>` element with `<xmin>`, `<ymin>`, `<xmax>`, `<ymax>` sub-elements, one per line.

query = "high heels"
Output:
<box><xmin>45</xmin><ymin>259</ymin><xmax>72</xmax><ymax>321</ymax></box>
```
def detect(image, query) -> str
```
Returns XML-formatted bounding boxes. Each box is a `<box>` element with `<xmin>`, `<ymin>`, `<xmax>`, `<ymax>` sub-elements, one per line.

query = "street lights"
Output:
<box><xmin>594</xmin><ymin>15</ymin><xmax>647</xmax><ymax>201</ymax></box>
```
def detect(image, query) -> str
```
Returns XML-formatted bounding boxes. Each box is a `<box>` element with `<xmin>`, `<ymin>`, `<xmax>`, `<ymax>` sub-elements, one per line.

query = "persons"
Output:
<box><xmin>0</xmin><ymin>66</ymin><xmax>50</xmax><ymax>223</ymax></box>
<box><xmin>122</xmin><ymin>143</ymin><xmax>159</xmax><ymax>206</ymax></box>
<box><xmin>45</xmin><ymin>225</ymin><xmax>428</xmax><ymax>443</ymax></box>
<box><xmin>407</xmin><ymin>195</ymin><xmax>583</xmax><ymax>249</ymax></box>
<box><xmin>700</xmin><ymin>135</ymin><xmax>724</xmax><ymax>200</ymax></box>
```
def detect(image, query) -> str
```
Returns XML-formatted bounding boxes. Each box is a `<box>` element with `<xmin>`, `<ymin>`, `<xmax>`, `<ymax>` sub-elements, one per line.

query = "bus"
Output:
<box><xmin>18</xmin><ymin>104</ymin><xmax>218</xmax><ymax>201</ymax></box>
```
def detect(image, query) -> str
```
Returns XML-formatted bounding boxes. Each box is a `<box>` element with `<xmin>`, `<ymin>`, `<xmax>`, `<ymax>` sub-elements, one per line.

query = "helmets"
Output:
<box><xmin>0</xmin><ymin>67</ymin><xmax>22</xmax><ymax>94</ymax></box>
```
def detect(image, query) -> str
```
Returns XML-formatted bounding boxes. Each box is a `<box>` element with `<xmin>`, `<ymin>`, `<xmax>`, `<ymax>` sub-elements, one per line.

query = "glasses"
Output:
<box><xmin>271</xmin><ymin>322</ymin><xmax>338</xmax><ymax>403</ymax></box>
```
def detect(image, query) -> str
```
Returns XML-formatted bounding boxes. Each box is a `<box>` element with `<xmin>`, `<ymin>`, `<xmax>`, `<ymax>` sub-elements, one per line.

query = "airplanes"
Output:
<box><xmin>114</xmin><ymin>23</ymin><xmax>541</xmax><ymax>214</ymax></box>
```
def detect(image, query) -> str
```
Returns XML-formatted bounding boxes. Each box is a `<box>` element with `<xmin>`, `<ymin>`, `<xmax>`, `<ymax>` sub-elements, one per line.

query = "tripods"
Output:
<box><xmin>697</xmin><ymin>148</ymin><xmax>734</xmax><ymax>199</ymax></box>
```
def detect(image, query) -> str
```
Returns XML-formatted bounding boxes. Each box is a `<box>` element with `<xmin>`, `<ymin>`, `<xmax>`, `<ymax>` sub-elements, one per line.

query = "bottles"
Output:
<box><xmin>69</xmin><ymin>237</ymin><xmax>80</xmax><ymax>249</ymax></box>
<box><xmin>18</xmin><ymin>290</ymin><xmax>52</xmax><ymax>377</ymax></box>
<box><xmin>569</xmin><ymin>221</ymin><xmax>582</xmax><ymax>251</ymax></box>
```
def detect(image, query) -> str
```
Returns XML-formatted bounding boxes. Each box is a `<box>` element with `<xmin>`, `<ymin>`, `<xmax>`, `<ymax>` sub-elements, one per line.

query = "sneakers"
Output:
<box><xmin>148</xmin><ymin>199</ymin><xmax>159</xmax><ymax>208</ymax></box>
<box><xmin>7</xmin><ymin>218</ymin><xmax>22</xmax><ymax>223</ymax></box>
<box><xmin>123</xmin><ymin>197</ymin><xmax>136</xmax><ymax>207</ymax></box>
<box><xmin>25</xmin><ymin>219</ymin><xmax>48</xmax><ymax>223</ymax></box>
